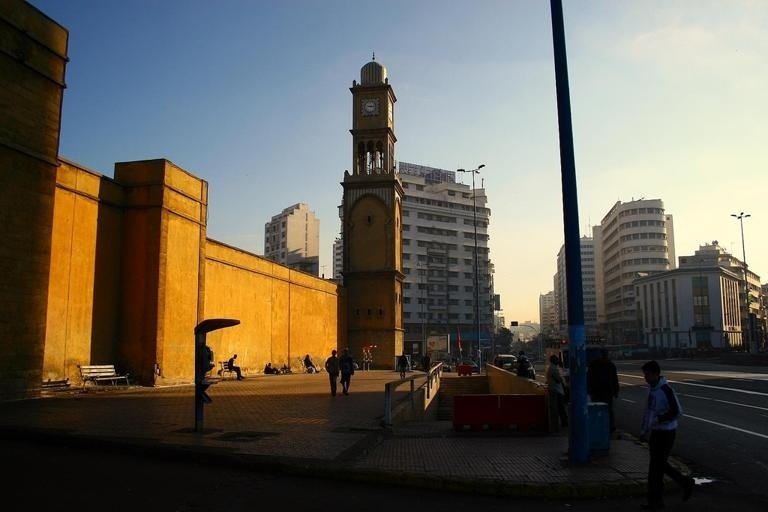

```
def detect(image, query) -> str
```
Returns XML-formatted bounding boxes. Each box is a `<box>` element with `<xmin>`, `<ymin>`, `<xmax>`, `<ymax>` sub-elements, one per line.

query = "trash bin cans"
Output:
<box><xmin>589</xmin><ymin>402</ymin><xmax>609</xmax><ymax>442</ymax></box>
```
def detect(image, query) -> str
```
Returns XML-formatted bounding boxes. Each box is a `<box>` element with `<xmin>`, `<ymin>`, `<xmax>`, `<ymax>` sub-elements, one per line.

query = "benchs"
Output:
<box><xmin>219</xmin><ymin>361</ymin><xmax>236</xmax><ymax>380</ymax></box>
<box><xmin>76</xmin><ymin>363</ymin><xmax>129</xmax><ymax>394</ymax></box>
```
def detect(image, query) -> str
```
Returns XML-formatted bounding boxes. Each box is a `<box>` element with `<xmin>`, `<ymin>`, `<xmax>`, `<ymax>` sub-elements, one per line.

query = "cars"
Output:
<box><xmin>441</xmin><ymin>359</ymin><xmax>479</xmax><ymax>373</ymax></box>
<box><xmin>511</xmin><ymin>360</ymin><xmax>536</xmax><ymax>380</ymax></box>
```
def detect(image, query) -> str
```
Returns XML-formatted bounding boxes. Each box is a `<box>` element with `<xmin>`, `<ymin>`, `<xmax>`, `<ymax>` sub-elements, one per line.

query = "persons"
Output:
<box><xmin>338</xmin><ymin>347</ymin><xmax>355</xmax><ymax>396</ymax></box>
<box><xmin>325</xmin><ymin>350</ymin><xmax>340</xmax><ymax>398</ymax></box>
<box><xmin>265</xmin><ymin>362</ymin><xmax>280</xmax><ymax>375</ymax></box>
<box><xmin>585</xmin><ymin>344</ymin><xmax>620</xmax><ymax>439</ymax></box>
<box><xmin>515</xmin><ymin>350</ymin><xmax>537</xmax><ymax>380</ymax></box>
<box><xmin>420</xmin><ymin>353</ymin><xmax>431</xmax><ymax>374</ymax></box>
<box><xmin>545</xmin><ymin>355</ymin><xmax>569</xmax><ymax>431</ymax></box>
<box><xmin>228</xmin><ymin>354</ymin><xmax>245</xmax><ymax>381</ymax></box>
<box><xmin>638</xmin><ymin>357</ymin><xmax>694</xmax><ymax>509</ymax></box>
<box><xmin>397</xmin><ymin>352</ymin><xmax>410</xmax><ymax>379</ymax></box>
<box><xmin>304</xmin><ymin>354</ymin><xmax>320</xmax><ymax>374</ymax></box>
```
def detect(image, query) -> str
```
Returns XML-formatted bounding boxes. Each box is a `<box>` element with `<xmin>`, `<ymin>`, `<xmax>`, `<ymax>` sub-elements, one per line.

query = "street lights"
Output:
<box><xmin>731</xmin><ymin>212</ymin><xmax>751</xmax><ymax>319</ymax></box>
<box><xmin>457</xmin><ymin>165</ymin><xmax>486</xmax><ymax>374</ymax></box>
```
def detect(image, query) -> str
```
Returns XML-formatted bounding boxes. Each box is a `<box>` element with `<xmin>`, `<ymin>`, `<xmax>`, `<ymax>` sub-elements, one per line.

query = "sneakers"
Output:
<box><xmin>639</xmin><ymin>503</ymin><xmax>665</xmax><ymax>509</ymax></box>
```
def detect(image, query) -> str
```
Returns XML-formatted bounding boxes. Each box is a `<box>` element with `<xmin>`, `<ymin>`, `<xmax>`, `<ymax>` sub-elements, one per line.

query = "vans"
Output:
<box><xmin>544</xmin><ymin>338</ymin><xmax>604</xmax><ymax>389</ymax></box>
<box><xmin>493</xmin><ymin>354</ymin><xmax>516</xmax><ymax>369</ymax></box>
